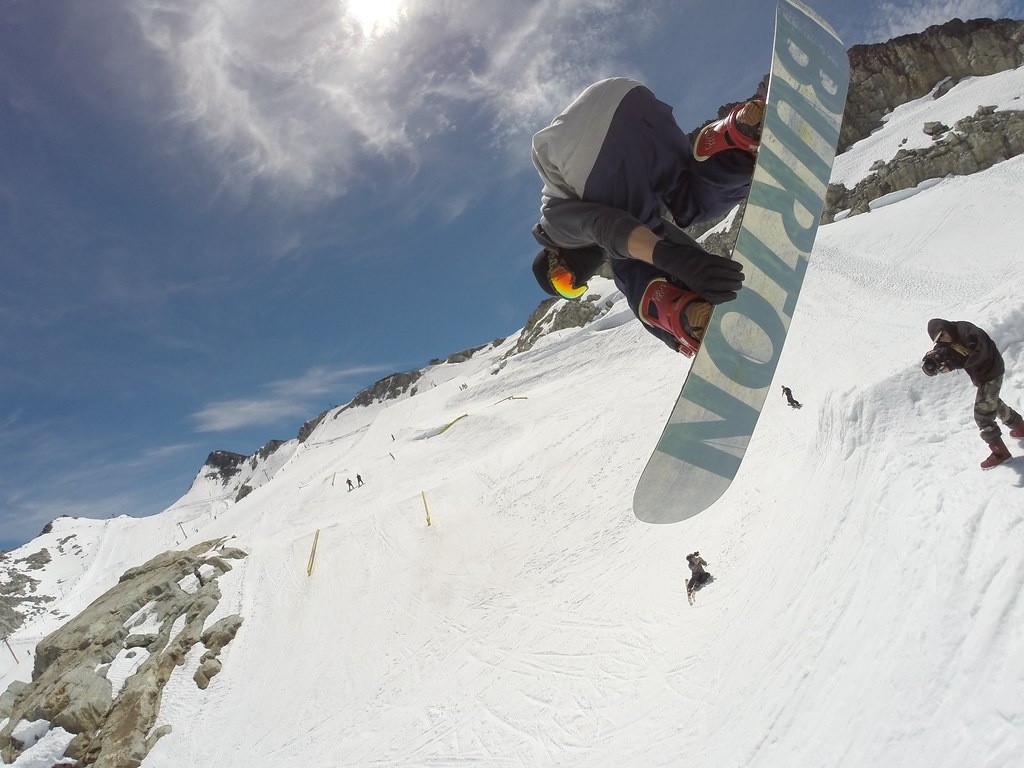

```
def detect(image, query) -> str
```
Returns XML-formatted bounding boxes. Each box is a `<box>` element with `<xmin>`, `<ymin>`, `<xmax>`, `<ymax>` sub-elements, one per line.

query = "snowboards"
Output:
<box><xmin>632</xmin><ymin>1</ymin><xmax>850</xmax><ymax>525</ymax></box>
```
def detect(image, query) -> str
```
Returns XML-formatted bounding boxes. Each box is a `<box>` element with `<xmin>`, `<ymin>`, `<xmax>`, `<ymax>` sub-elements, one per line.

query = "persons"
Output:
<box><xmin>356</xmin><ymin>474</ymin><xmax>363</xmax><ymax>487</ymax></box>
<box><xmin>686</xmin><ymin>552</ymin><xmax>710</xmax><ymax>595</ymax></box>
<box><xmin>781</xmin><ymin>385</ymin><xmax>799</xmax><ymax>405</ymax></box>
<box><xmin>389</xmin><ymin>453</ymin><xmax>395</xmax><ymax>460</ymax></box>
<box><xmin>391</xmin><ymin>434</ymin><xmax>395</xmax><ymax>441</ymax></box>
<box><xmin>531</xmin><ymin>77</ymin><xmax>763</xmax><ymax>357</ymax></box>
<box><xmin>346</xmin><ymin>478</ymin><xmax>354</xmax><ymax>492</ymax></box>
<box><xmin>926</xmin><ymin>318</ymin><xmax>1024</xmax><ymax>470</ymax></box>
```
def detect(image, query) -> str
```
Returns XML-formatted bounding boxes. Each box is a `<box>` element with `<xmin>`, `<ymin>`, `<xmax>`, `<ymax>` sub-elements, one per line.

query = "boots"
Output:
<box><xmin>980</xmin><ymin>439</ymin><xmax>1013</xmax><ymax>469</ymax></box>
<box><xmin>1009</xmin><ymin>420</ymin><xmax>1024</xmax><ymax>437</ymax></box>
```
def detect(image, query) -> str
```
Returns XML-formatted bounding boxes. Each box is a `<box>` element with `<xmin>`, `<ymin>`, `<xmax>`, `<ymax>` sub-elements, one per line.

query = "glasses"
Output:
<box><xmin>548</xmin><ymin>256</ymin><xmax>589</xmax><ymax>301</ymax></box>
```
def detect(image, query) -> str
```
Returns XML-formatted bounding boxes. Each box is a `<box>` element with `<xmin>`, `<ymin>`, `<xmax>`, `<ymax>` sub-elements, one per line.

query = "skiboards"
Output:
<box><xmin>685</xmin><ymin>577</ymin><xmax>696</xmax><ymax>605</ymax></box>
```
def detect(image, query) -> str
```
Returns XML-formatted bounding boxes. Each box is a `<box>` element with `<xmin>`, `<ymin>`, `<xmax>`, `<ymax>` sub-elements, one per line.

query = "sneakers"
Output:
<box><xmin>637</xmin><ymin>276</ymin><xmax>711</xmax><ymax>348</ymax></box>
<box><xmin>694</xmin><ymin>99</ymin><xmax>766</xmax><ymax>162</ymax></box>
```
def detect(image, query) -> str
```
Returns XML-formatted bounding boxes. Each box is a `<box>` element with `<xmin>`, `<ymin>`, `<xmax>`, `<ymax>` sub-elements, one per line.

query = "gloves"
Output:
<box><xmin>652</xmin><ymin>240</ymin><xmax>745</xmax><ymax>304</ymax></box>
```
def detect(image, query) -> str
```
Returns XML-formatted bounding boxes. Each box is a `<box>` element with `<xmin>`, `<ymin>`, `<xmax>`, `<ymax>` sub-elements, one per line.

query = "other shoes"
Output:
<box><xmin>687</xmin><ymin>587</ymin><xmax>691</xmax><ymax>593</ymax></box>
<box><xmin>694</xmin><ymin>582</ymin><xmax>699</xmax><ymax>588</ymax></box>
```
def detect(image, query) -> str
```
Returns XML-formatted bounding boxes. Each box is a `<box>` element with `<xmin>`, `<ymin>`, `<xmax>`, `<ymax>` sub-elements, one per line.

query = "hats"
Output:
<box><xmin>933</xmin><ymin>330</ymin><xmax>943</xmax><ymax>345</ymax></box>
<box><xmin>532</xmin><ymin>249</ymin><xmax>562</xmax><ymax>296</ymax></box>
